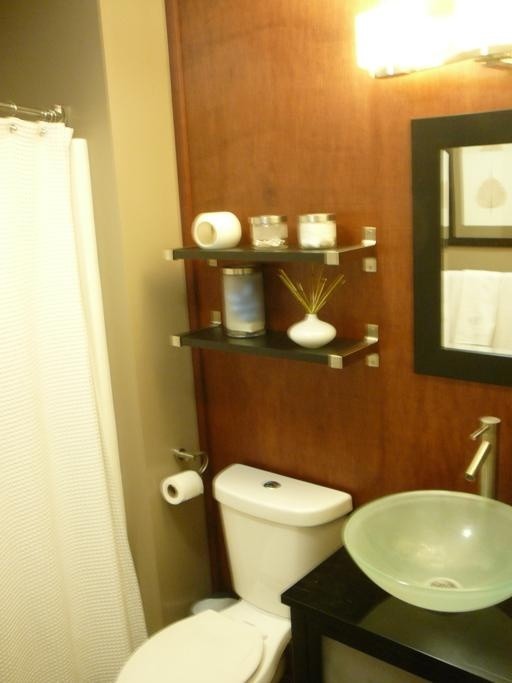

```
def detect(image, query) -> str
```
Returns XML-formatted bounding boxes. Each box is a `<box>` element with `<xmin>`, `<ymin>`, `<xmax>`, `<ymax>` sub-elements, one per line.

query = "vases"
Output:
<box><xmin>287</xmin><ymin>313</ymin><xmax>337</xmax><ymax>349</ymax></box>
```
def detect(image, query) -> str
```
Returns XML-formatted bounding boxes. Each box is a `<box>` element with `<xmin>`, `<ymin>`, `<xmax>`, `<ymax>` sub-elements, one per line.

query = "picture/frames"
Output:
<box><xmin>444</xmin><ymin>145</ymin><xmax>512</xmax><ymax>248</ymax></box>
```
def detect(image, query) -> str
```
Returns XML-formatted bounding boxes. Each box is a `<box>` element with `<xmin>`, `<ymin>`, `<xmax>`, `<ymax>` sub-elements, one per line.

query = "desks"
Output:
<box><xmin>281</xmin><ymin>549</ymin><xmax>511</xmax><ymax>683</ymax></box>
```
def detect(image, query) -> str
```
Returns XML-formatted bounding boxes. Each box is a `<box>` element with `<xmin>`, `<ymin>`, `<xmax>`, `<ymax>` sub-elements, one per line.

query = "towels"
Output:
<box><xmin>441</xmin><ymin>271</ymin><xmax>512</xmax><ymax>359</ymax></box>
<box><xmin>453</xmin><ymin>268</ymin><xmax>501</xmax><ymax>346</ymax></box>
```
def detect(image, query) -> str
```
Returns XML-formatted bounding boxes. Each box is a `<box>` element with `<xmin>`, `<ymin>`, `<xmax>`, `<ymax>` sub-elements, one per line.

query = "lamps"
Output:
<box><xmin>354</xmin><ymin>1</ymin><xmax>512</xmax><ymax>79</ymax></box>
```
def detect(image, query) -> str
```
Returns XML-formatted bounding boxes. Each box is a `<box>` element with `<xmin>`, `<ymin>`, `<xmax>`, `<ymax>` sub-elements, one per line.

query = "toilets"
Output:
<box><xmin>117</xmin><ymin>461</ymin><xmax>354</xmax><ymax>683</ymax></box>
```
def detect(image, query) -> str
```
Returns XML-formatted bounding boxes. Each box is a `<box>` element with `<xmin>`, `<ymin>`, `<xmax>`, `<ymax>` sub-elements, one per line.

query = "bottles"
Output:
<box><xmin>298</xmin><ymin>212</ymin><xmax>337</xmax><ymax>250</ymax></box>
<box><xmin>248</xmin><ymin>213</ymin><xmax>288</xmax><ymax>249</ymax></box>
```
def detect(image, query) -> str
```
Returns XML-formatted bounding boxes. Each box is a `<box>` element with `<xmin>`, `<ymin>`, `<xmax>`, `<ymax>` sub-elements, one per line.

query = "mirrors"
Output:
<box><xmin>411</xmin><ymin>113</ymin><xmax>512</xmax><ymax>388</ymax></box>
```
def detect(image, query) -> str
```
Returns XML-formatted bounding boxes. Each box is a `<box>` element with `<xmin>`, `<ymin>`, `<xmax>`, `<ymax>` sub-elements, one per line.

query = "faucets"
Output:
<box><xmin>464</xmin><ymin>412</ymin><xmax>502</xmax><ymax>497</ymax></box>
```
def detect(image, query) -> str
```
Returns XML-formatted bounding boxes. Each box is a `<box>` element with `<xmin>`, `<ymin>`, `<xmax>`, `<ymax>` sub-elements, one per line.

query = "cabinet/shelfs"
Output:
<box><xmin>163</xmin><ymin>226</ymin><xmax>380</xmax><ymax>369</ymax></box>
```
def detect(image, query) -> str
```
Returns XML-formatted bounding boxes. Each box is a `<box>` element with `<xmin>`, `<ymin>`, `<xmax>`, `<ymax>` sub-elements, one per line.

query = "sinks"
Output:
<box><xmin>341</xmin><ymin>486</ymin><xmax>512</xmax><ymax>615</ymax></box>
